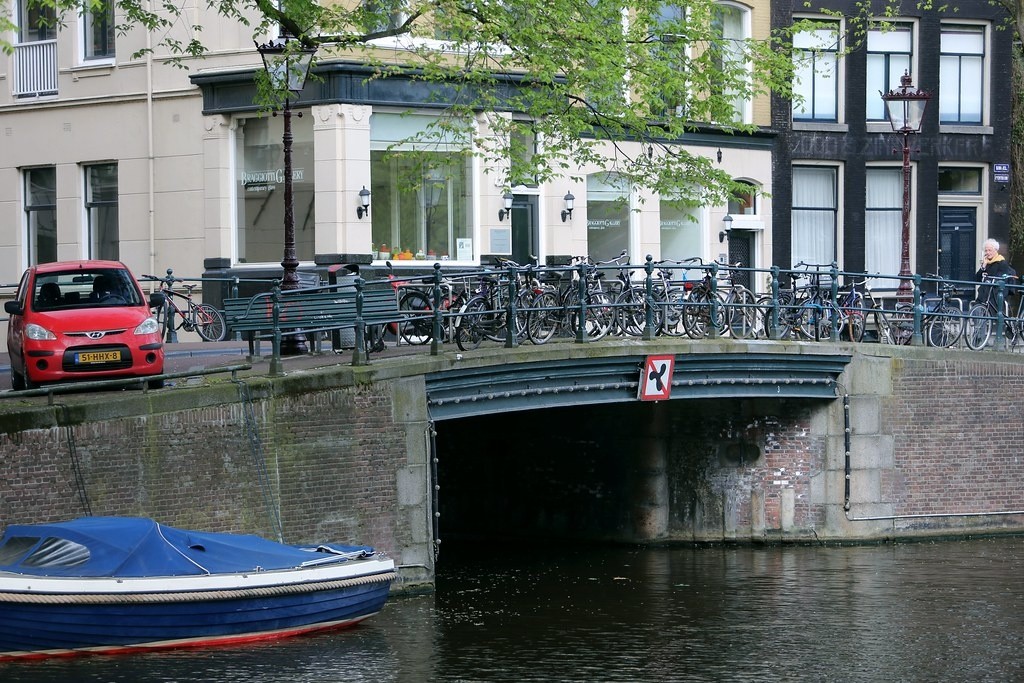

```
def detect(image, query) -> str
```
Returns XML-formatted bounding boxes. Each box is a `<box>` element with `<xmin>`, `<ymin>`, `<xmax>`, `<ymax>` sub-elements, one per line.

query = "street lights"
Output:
<box><xmin>879</xmin><ymin>68</ymin><xmax>937</xmax><ymax>338</ymax></box>
<box><xmin>253</xmin><ymin>20</ymin><xmax>318</xmax><ymax>355</ymax></box>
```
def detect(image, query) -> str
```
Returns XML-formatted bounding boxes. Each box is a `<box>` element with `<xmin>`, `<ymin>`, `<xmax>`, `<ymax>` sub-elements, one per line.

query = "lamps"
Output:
<box><xmin>356</xmin><ymin>186</ymin><xmax>370</xmax><ymax>219</ymax></box>
<box><xmin>561</xmin><ymin>190</ymin><xmax>576</xmax><ymax>222</ymax></box>
<box><xmin>719</xmin><ymin>213</ymin><xmax>733</xmax><ymax>243</ymax></box>
<box><xmin>499</xmin><ymin>191</ymin><xmax>514</xmax><ymax>222</ymax></box>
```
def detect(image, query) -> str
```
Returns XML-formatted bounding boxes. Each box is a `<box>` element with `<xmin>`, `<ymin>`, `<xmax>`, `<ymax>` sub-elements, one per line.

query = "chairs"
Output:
<box><xmin>38</xmin><ymin>283</ymin><xmax>62</xmax><ymax>306</ymax></box>
<box><xmin>91</xmin><ymin>276</ymin><xmax>114</xmax><ymax>299</ymax></box>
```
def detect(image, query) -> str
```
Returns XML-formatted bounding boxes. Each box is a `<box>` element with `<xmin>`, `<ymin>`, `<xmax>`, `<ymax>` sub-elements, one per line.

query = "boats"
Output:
<box><xmin>0</xmin><ymin>515</ymin><xmax>396</xmax><ymax>661</ymax></box>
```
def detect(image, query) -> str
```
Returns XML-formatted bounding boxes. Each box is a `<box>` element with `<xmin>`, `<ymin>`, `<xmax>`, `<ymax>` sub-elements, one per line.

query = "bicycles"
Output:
<box><xmin>142</xmin><ymin>273</ymin><xmax>226</xmax><ymax>344</ymax></box>
<box><xmin>397</xmin><ymin>250</ymin><xmax>1024</xmax><ymax>351</ymax></box>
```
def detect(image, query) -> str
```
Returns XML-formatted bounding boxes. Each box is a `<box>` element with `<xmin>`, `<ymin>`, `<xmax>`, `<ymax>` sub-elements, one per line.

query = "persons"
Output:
<box><xmin>974</xmin><ymin>239</ymin><xmax>1008</xmax><ymax>347</ymax></box>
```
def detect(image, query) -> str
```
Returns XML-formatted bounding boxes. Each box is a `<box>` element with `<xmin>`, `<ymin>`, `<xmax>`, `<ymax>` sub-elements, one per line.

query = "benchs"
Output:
<box><xmin>224</xmin><ymin>290</ymin><xmax>405</xmax><ymax>357</ymax></box>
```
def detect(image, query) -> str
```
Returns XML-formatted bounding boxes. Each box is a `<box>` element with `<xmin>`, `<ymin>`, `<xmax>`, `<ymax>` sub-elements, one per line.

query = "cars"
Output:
<box><xmin>3</xmin><ymin>260</ymin><xmax>166</xmax><ymax>390</ymax></box>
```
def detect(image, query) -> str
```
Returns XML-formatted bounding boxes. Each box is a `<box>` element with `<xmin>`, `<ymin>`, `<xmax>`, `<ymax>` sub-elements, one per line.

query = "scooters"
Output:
<box><xmin>365</xmin><ymin>260</ymin><xmax>462</xmax><ymax>346</ymax></box>
